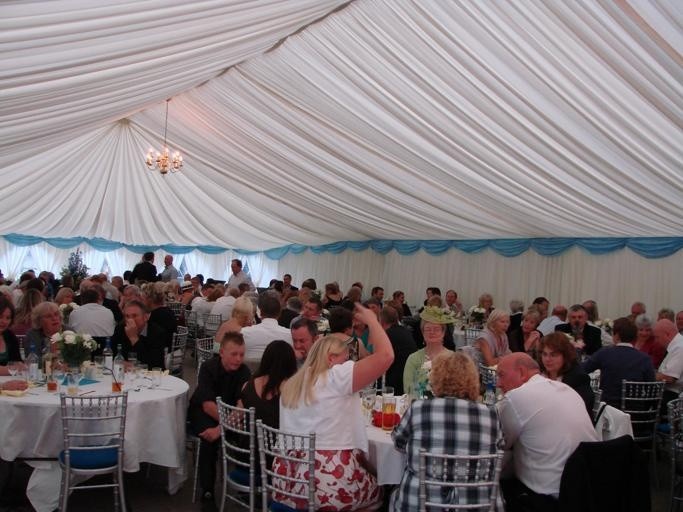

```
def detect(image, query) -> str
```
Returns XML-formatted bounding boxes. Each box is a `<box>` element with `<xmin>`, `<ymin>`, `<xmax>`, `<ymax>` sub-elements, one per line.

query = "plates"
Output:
<box><xmin>0</xmin><ymin>379</ymin><xmax>31</xmax><ymax>396</ymax></box>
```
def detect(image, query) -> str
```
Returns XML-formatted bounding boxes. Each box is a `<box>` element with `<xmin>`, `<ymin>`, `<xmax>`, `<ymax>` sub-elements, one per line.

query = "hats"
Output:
<box><xmin>180</xmin><ymin>280</ymin><xmax>193</xmax><ymax>290</ymax></box>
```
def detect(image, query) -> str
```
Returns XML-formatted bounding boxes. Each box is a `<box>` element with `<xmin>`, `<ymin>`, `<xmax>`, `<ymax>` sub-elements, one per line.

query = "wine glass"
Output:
<box><xmin>7</xmin><ymin>361</ymin><xmax>18</xmax><ymax>376</ymax></box>
<box><xmin>363</xmin><ymin>395</ymin><xmax>376</xmax><ymax>426</ymax></box>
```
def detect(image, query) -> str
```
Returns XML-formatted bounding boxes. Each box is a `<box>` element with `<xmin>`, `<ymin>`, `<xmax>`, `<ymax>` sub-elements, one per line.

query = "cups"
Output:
<box><xmin>381</xmin><ymin>397</ymin><xmax>396</xmax><ymax>429</ymax></box>
<box><xmin>127</xmin><ymin>353</ymin><xmax>169</xmax><ymax>390</ymax></box>
<box><xmin>382</xmin><ymin>387</ymin><xmax>394</xmax><ymax>398</ymax></box>
<box><xmin>22</xmin><ymin>368</ymin><xmax>30</xmax><ymax>378</ymax></box>
<box><xmin>67</xmin><ymin>374</ymin><xmax>79</xmax><ymax>396</ymax></box>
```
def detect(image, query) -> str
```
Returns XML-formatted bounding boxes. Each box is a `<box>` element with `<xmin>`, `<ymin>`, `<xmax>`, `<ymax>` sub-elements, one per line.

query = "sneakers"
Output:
<box><xmin>200</xmin><ymin>487</ymin><xmax>218</xmax><ymax>512</ymax></box>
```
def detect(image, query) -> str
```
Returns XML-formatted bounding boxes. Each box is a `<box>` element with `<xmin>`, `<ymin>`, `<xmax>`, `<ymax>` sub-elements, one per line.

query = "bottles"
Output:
<box><xmin>41</xmin><ymin>338</ymin><xmax>53</xmax><ymax>378</ymax></box>
<box><xmin>102</xmin><ymin>337</ymin><xmax>112</xmax><ymax>374</ymax></box>
<box><xmin>47</xmin><ymin>341</ymin><xmax>58</xmax><ymax>391</ymax></box>
<box><xmin>26</xmin><ymin>344</ymin><xmax>38</xmax><ymax>386</ymax></box>
<box><xmin>112</xmin><ymin>343</ymin><xmax>124</xmax><ymax>391</ymax></box>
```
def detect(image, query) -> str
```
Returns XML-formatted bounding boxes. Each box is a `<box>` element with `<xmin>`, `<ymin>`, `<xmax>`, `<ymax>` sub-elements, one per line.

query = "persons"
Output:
<box><xmin>183</xmin><ymin>329</ymin><xmax>254</xmax><ymax>511</ymax></box>
<box><xmin>1</xmin><ymin>269</ymin><xmax>682</xmax><ymax>436</ymax></box>
<box><xmin>492</xmin><ymin>349</ymin><xmax>603</xmax><ymax>511</ymax></box>
<box><xmin>227</xmin><ymin>259</ymin><xmax>255</xmax><ymax>290</ymax></box>
<box><xmin>387</xmin><ymin>352</ymin><xmax>508</xmax><ymax>512</ymax></box>
<box><xmin>199</xmin><ymin>341</ymin><xmax>296</xmax><ymax>512</ymax></box>
<box><xmin>159</xmin><ymin>254</ymin><xmax>184</xmax><ymax>280</ymax></box>
<box><xmin>269</xmin><ymin>301</ymin><xmax>396</xmax><ymax>512</ymax></box>
<box><xmin>131</xmin><ymin>251</ymin><xmax>161</xmax><ymax>281</ymax></box>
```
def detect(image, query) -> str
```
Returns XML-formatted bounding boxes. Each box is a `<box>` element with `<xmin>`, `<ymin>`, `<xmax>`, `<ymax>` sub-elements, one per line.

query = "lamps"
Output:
<box><xmin>140</xmin><ymin>100</ymin><xmax>184</xmax><ymax>177</ymax></box>
